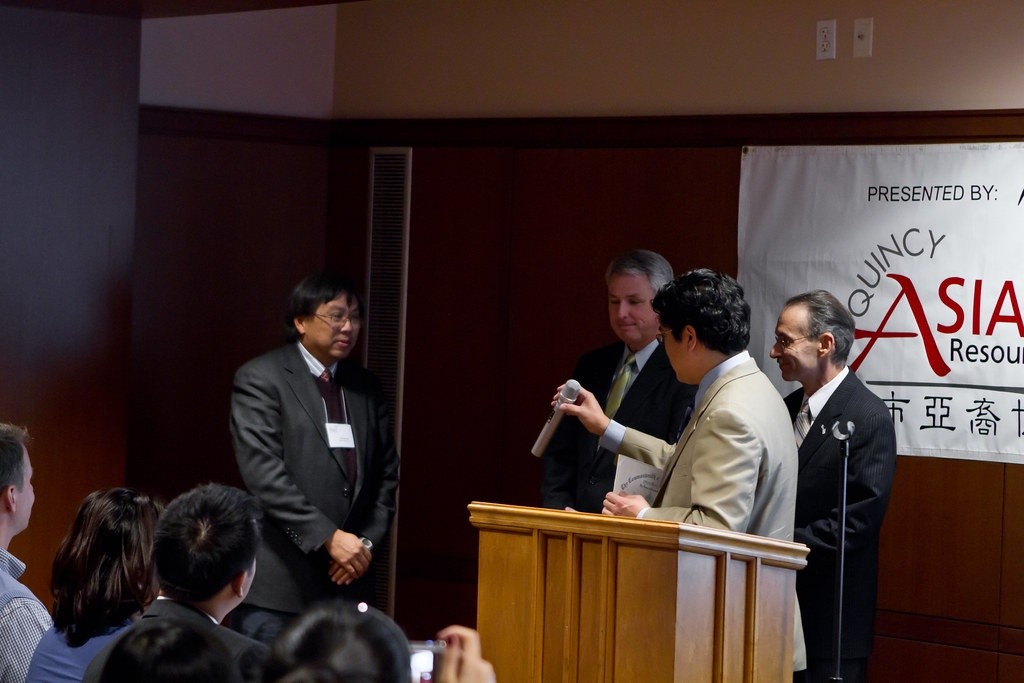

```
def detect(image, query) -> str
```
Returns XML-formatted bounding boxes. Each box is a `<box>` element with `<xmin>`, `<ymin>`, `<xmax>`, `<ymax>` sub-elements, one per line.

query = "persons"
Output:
<box><xmin>268</xmin><ymin>605</ymin><xmax>496</xmax><ymax>683</ymax></box>
<box><xmin>768</xmin><ymin>290</ymin><xmax>896</xmax><ymax>683</ymax></box>
<box><xmin>539</xmin><ymin>248</ymin><xmax>697</xmax><ymax>511</ymax></box>
<box><xmin>82</xmin><ymin>485</ymin><xmax>273</xmax><ymax>683</ymax></box>
<box><xmin>231</xmin><ymin>271</ymin><xmax>399</xmax><ymax>642</ymax></box>
<box><xmin>0</xmin><ymin>423</ymin><xmax>55</xmax><ymax>682</ymax></box>
<box><xmin>552</xmin><ymin>269</ymin><xmax>807</xmax><ymax>672</ymax></box>
<box><xmin>27</xmin><ymin>487</ymin><xmax>161</xmax><ymax>683</ymax></box>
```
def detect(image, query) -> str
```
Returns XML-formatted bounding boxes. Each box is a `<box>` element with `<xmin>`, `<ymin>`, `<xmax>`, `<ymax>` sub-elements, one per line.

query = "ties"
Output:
<box><xmin>317</xmin><ymin>367</ymin><xmax>356</xmax><ymax>496</ymax></box>
<box><xmin>794</xmin><ymin>399</ymin><xmax>812</xmax><ymax>452</ymax></box>
<box><xmin>605</xmin><ymin>354</ymin><xmax>636</xmax><ymax>418</ymax></box>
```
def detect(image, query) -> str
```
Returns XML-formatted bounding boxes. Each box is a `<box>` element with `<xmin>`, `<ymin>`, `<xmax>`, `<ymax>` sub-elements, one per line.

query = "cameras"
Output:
<box><xmin>405</xmin><ymin>640</ymin><xmax>448</xmax><ymax>683</ymax></box>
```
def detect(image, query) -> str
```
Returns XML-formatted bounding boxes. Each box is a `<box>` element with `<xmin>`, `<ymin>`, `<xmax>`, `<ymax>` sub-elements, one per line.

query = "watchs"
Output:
<box><xmin>361</xmin><ymin>537</ymin><xmax>374</xmax><ymax>550</ymax></box>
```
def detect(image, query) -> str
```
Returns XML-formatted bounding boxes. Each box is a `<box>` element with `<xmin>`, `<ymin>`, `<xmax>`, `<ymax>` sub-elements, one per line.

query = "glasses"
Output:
<box><xmin>306</xmin><ymin>311</ymin><xmax>363</xmax><ymax>329</ymax></box>
<box><xmin>775</xmin><ymin>334</ymin><xmax>816</xmax><ymax>349</ymax></box>
<box><xmin>655</xmin><ymin>330</ymin><xmax>680</xmax><ymax>348</ymax></box>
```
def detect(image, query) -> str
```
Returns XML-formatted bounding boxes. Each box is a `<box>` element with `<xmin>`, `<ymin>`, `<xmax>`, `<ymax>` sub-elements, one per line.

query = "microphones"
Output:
<box><xmin>531</xmin><ymin>379</ymin><xmax>581</xmax><ymax>457</ymax></box>
